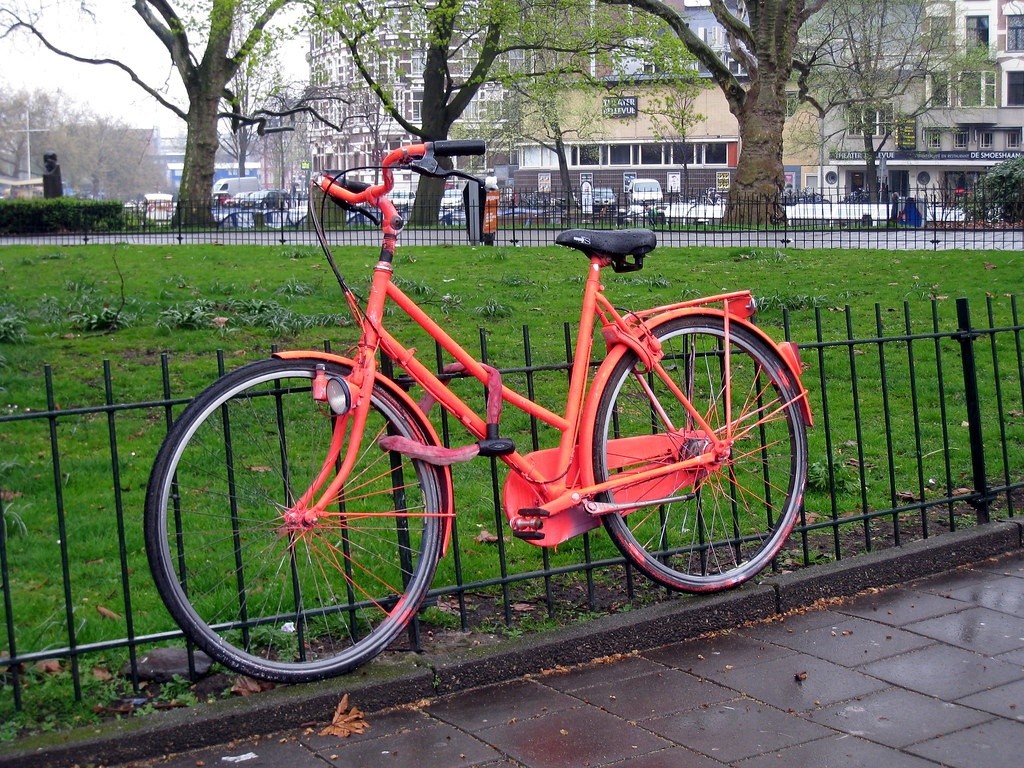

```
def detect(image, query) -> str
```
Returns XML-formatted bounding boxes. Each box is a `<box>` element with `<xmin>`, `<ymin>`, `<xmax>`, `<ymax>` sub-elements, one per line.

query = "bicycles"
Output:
<box><xmin>143</xmin><ymin>138</ymin><xmax>815</xmax><ymax>680</ymax></box>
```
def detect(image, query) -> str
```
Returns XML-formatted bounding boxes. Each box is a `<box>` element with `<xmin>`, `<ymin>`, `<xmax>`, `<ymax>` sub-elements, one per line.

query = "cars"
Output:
<box><xmin>211</xmin><ymin>188</ymin><xmax>308</xmax><ymax>215</ymax></box>
<box><xmin>390</xmin><ymin>191</ymin><xmax>415</xmax><ymax>209</ymax></box>
<box><xmin>440</xmin><ymin>189</ymin><xmax>465</xmax><ymax>209</ymax></box>
<box><xmin>591</xmin><ymin>188</ymin><xmax>617</xmax><ymax>212</ymax></box>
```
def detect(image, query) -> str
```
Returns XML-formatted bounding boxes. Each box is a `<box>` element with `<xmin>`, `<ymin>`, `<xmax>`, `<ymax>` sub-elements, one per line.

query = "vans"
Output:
<box><xmin>213</xmin><ymin>177</ymin><xmax>261</xmax><ymax>197</ymax></box>
<box><xmin>144</xmin><ymin>192</ymin><xmax>174</xmax><ymax>226</ymax></box>
<box><xmin>624</xmin><ymin>178</ymin><xmax>663</xmax><ymax>206</ymax></box>
